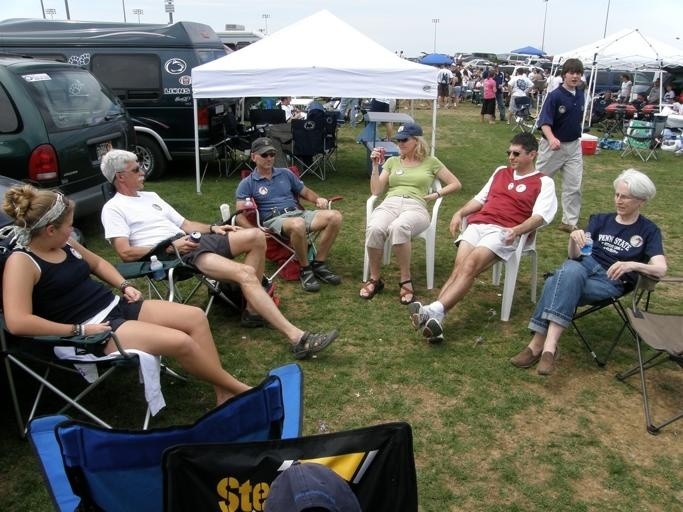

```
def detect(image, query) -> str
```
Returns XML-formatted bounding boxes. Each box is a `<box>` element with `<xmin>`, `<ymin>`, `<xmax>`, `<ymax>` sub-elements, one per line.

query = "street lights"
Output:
<box><xmin>164</xmin><ymin>0</ymin><xmax>175</xmax><ymax>24</ymax></box>
<box><xmin>541</xmin><ymin>0</ymin><xmax>549</xmax><ymax>53</ymax></box>
<box><xmin>431</xmin><ymin>18</ymin><xmax>440</xmax><ymax>52</ymax></box>
<box><xmin>260</xmin><ymin>14</ymin><xmax>271</xmax><ymax>33</ymax></box>
<box><xmin>44</xmin><ymin>9</ymin><xmax>56</xmax><ymax>19</ymax></box>
<box><xmin>132</xmin><ymin>8</ymin><xmax>143</xmax><ymax>24</ymax></box>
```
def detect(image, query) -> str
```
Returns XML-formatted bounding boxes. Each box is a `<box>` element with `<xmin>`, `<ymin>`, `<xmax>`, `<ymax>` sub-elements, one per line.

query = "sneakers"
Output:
<box><xmin>422</xmin><ymin>317</ymin><xmax>444</xmax><ymax>345</ymax></box>
<box><xmin>537</xmin><ymin>346</ymin><xmax>558</xmax><ymax>375</ymax></box>
<box><xmin>510</xmin><ymin>346</ymin><xmax>543</xmax><ymax>369</ymax></box>
<box><xmin>409</xmin><ymin>301</ymin><xmax>434</xmax><ymax>332</ymax></box>
<box><xmin>298</xmin><ymin>266</ymin><xmax>322</xmax><ymax>292</ymax></box>
<box><xmin>558</xmin><ymin>222</ymin><xmax>580</xmax><ymax>234</ymax></box>
<box><xmin>311</xmin><ymin>265</ymin><xmax>341</xmax><ymax>286</ymax></box>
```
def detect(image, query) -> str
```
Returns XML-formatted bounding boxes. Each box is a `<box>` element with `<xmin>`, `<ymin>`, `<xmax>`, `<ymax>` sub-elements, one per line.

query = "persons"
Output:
<box><xmin>277</xmin><ymin>97</ymin><xmax>396</xmax><ymax>141</ymax></box>
<box><xmin>407</xmin><ymin>132</ymin><xmax>558</xmax><ymax>344</ymax></box>
<box><xmin>593</xmin><ymin>73</ymin><xmax>676</xmax><ymax>123</ymax></box>
<box><xmin>2</xmin><ymin>184</ymin><xmax>253</xmax><ymax>408</ymax></box>
<box><xmin>99</xmin><ymin>149</ymin><xmax>338</xmax><ymax>361</ymax></box>
<box><xmin>509</xmin><ymin>168</ymin><xmax>668</xmax><ymax>376</ymax></box>
<box><xmin>536</xmin><ymin>58</ymin><xmax>585</xmax><ymax>232</ymax></box>
<box><xmin>436</xmin><ymin>63</ymin><xmax>551</xmax><ymax>125</ymax></box>
<box><xmin>358</xmin><ymin>121</ymin><xmax>463</xmax><ymax>304</ymax></box>
<box><xmin>235</xmin><ymin>137</ymin><xmax>343</xmax><ymax>292</ymax></box>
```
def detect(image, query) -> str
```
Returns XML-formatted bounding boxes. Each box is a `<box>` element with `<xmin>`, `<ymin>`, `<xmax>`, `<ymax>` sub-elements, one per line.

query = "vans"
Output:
<box><xmin>216</xmin><ymin>28</ymin><xmax>264</xmax><ymax>51</ymax></box>
<box><xmin>0</xmin><ymin>16</ymin><xmax>237</xmax><ymax>180</ymax></box>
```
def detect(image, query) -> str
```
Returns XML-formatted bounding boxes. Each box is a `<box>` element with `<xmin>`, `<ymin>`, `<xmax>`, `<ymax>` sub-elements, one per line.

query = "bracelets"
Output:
<box><xmin>210</xmin><ymin>224</ymin><xmax>215</xmax><ymax>233</ymax></box>
<box><xmin>119</xmin><ymin>280</ymin><xmax>135</xmax><ymax>294</ymax></box>
<box><xmin>431</xmin><ymin>191</ymin><xmax>440</xmax><ymax>197</ymax></box>
<box><xmin>71</xmin><ymin>323</ymin><xmax>86</xmax><ymax>337</ymax></box>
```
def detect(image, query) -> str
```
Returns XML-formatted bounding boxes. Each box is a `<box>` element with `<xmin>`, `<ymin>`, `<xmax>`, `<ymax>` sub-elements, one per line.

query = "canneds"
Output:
<box><xmin>188</xmin><ymin>231</ymin><xmax>202</xmax><ymax>243</ymax></box>
<box><xmin>374</xmin><ymin>147</ymin><xmax>386</xmax><ymax>165</ymax></box>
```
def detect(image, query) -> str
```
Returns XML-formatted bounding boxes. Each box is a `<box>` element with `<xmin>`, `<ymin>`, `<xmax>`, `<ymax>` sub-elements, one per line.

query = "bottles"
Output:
<box><xmin>581</xmin><ymin>233</ymin><xmax>594</xmax><ymax>255</ymax></box>
<box><xmin>147</xmin><ymin>255</ymin><xmax>168</xmax><ymax>281</ymax></box>
<box><xmin>240</xmin><ymin>198</ymin><xmax>252</xmax><ymax>209</ymax></box>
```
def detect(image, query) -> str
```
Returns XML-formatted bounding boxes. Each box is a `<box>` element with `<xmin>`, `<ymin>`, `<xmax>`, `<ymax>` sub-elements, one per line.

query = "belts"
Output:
<box><xmin>262</xmin><ymin>206</ymin><xmax>305</xmax><ymax>222</ymax></box>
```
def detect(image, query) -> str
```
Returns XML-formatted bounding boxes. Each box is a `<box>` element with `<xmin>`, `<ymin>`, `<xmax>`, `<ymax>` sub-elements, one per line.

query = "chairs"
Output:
<box><xmin>616</xmin><ymin>274</ymin><xmax>683</xmax><ymax>436</ymax></box>
<box><xmin>161</xmin><ymin>421</ymin><xmax>417</xmax><ymax>511</ymax></box>
<box><xmin>198</xmin><ymin>108</ymin><xmax>339</xmax><ymax>186</ymax></box>
<box><xmin>542</xmin><ymin>270</ymin><xmax>660</xmax><ymax>368</ymax></box>
<box><xmin>620</xmin><ymin>115</ymin><xmax>668</xmax><ymax>163</ymax></box>
<box><xmin>362</xmin><ymin>176</ymin><xmax>443</xmax><ymax>291</ymax></box>
<box><xmin>240</xmin><ymin>166</ymin><xmax>344</xmax><ymax>291</ymax></box>
<box><xmin>511</xmin><ymin>96</ymin><xmax>538</xmax><ymax>134</ymax></box>
<box><xmin>457</xmin><ymin>214</ymin><xmax>539</xmax><ymax>322</ymax></box>
<box><xmin>138</xmin><ymin>208</ymin><xmax>243</xmax><ymax>317</ymax></box>
<box><xmin>0</xmin><ymin>259</ymin><xmax>189</xmax><ymax>434</ymax></box>
<box><xmin>25</xmin><ymin>361</ymin><xmax>305</xmax><ymax>512</ymax></box>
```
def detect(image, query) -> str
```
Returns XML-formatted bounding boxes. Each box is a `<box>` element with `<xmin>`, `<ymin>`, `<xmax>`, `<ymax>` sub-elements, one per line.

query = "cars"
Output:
<box><xmin>450</xmin><ymin>53</ymin><xmax>657</xmax><ymax>121</ymax></box>
<box><xmin>1</xmin><ymin>175</ymin><xmax>87</xmax><ymax>263</ymax></box>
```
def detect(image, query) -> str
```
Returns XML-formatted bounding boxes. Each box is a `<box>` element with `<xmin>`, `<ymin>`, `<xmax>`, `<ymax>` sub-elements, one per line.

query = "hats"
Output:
<box><xmin>395</xmin><ymin>122</ymin><xmax>423</xmax><ymax>140</ymax></box>
<box><xmin>251</xmin><ymin>136</ymin><xmax>276</xmax><ymax>155</ymax></box>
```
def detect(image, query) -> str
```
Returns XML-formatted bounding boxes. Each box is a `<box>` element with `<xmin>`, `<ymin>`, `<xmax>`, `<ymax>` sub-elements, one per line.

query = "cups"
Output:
<box><xmin>499</xmin><ymin>230</ymin><xmax>514</xmax><ymax>252</ymax></box>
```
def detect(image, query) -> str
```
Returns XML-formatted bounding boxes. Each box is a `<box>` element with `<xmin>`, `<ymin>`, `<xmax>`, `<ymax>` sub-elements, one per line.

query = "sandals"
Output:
<box><xmin>399</xmin><ymin>279</ymin><xmax>415</xmax><ymax>305</ymax></box>
<box><xmin>359</xmin><ymin>278</ymin><xmax>385</xmax><ymax>299</ymax></box>
<box><xmin>291</xmin><ymin>329</ymin><xmax>340</xmax><ymax>360</ymax></box>
<box><xmin>240</xmin><ymin>308</ymin><xmax>271</xmax><ymax>328</ymax></box>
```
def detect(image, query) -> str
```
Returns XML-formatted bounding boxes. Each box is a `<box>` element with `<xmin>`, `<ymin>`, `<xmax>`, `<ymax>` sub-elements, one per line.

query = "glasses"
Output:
<box><xmin>132</xmin><ymin>164</ymin><xmax>140</xmax><ymax>174</ymax></box>
<box><xmin>396</xmin><ymin>138</ymin><xmax>408</xmax><ymax>142</ymax></box>
<box><xmin>260</xmin><ymin>153</ymin><xmax>276</xmax><ymax>158</ymax></box>
<box><xmin>507</xmin><ymin>150</ymin><xmax>530</xmax><ymax>156</ymax></box>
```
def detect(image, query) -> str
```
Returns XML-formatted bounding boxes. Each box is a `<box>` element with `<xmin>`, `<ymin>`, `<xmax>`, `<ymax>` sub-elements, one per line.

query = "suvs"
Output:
<box><xmin>0</xmin><ymin>57</ymin><xmax>138</xmax><ymax>219</ymax></box>
